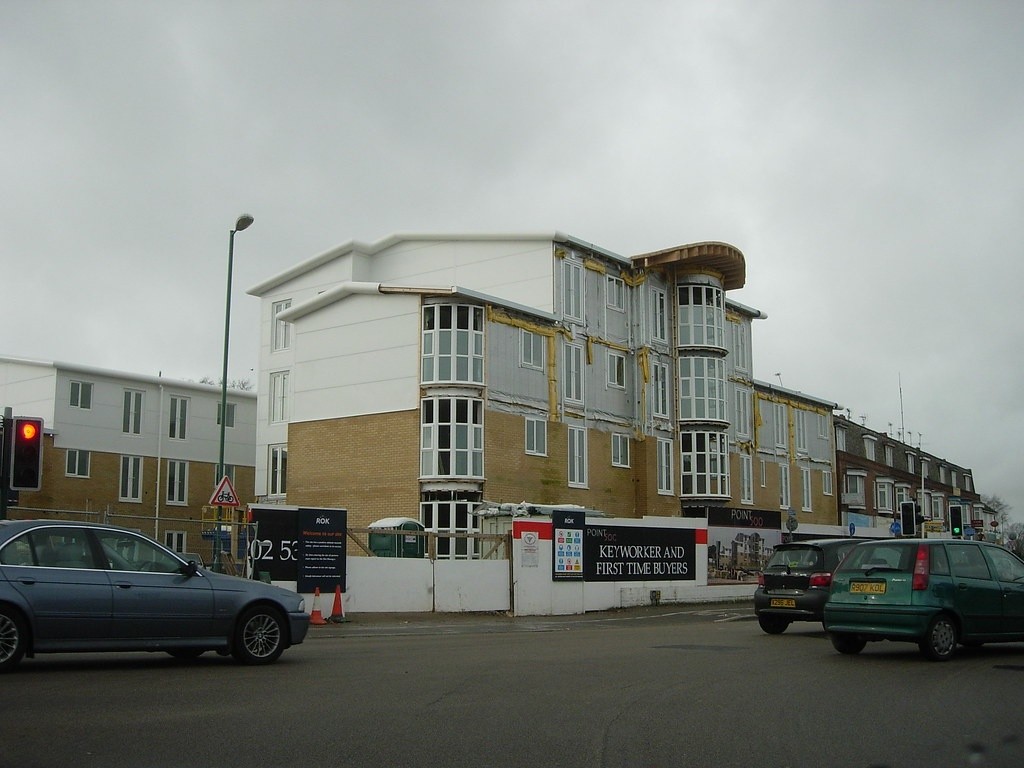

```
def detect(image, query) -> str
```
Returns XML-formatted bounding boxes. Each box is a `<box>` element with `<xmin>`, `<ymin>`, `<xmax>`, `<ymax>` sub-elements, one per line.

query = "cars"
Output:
<box><xmin>0</xmin><ymin>517</ymin><xmax>310</xmax><ymax>676</ymax></box>
<box><xmin>752</xmin><ymin>534</ymin><xmax>1024</xmax><ymax>658</ymax></box>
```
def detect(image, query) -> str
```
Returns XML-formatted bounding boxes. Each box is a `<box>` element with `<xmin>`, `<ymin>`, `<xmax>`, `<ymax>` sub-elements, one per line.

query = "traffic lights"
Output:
<box><xmin>899</xmin><ymin>501</ymin><xmax>918</xmax><ymax>537</ymax></box>
<box><xmin>8</xmin><ymin>413</ymin><xmax>46</xmax><ymax>493</ymax></box>
<box><xmin>948</xmin><ymin>504</ymin><xmax>964</xmax><ymax>538</ymax></box>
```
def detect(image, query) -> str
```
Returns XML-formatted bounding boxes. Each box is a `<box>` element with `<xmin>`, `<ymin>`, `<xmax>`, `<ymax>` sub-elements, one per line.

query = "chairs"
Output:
<box><xmin>953</xmin><ymin>564</ymin><xmax>974</xmax><ymax>577</ymax></box>
<box><xmin>57</xmin><ymin>543</ymin><xmax>88</xmax><ymax>569</ymax></box>
<box><xmin>28</xmin><ymin>545</ymin><xmax>49</xmax><ymax>567</ymax></box>
<box><xmin>871</xmin><ymin>558</ymin><xmax>887</xmax><ymax>565</ymax></box>
<box><xmin>788</xmin><ymin>552</ymin><xmax>801</xmax><ymax>562</ymax></box>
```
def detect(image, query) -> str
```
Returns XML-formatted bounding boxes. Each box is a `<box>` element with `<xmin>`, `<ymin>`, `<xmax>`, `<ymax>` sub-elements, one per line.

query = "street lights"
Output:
<box><xmin>211</xmin><ymin>209</ymin><xmax>257</xmax><ymax>574</ymax></box>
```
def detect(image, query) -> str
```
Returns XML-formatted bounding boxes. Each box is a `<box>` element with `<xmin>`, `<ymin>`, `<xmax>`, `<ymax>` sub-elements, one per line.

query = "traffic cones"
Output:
<box><xmin>309</xmin><ymin>581</ymin><xmax>347</xmax><ymax>625</ymax></box>
